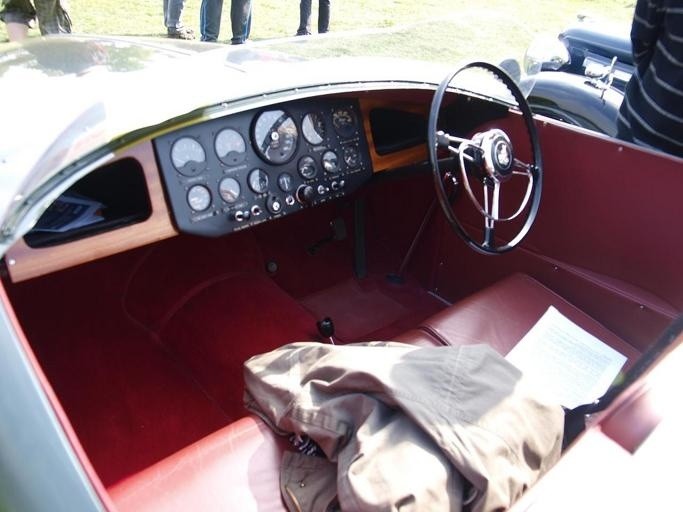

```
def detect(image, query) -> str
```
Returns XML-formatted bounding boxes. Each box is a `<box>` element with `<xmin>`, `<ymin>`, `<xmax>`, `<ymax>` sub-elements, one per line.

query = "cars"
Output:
<box><xmin>489</xmin><ymin>21</ymin><xmax>646</xmax><ymax>142</ymax></box>
<box><xmin>0</xmin><ymin>30</ymin><xmax>683</xmax><ymax>512</ymax></box>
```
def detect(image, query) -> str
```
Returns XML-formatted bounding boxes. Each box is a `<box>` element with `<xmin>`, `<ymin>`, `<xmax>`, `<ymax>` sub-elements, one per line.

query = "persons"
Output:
<box><xmin>162</xmin><ymin>1</ymin><xmax>195</xmax><ymax>42</ymax></box>
<box><xmin>0</xmin><ymin>1</ymin><xmax>72</xmax><ymax>42</ymax></box>
<box><xmin>612</xmin><ymin>0</ymin><xmax>682</xmax><ymax>161</ymax></box>
<box><xmin>295</xmin><ymin>0</ymin><xmax>330</xmax><ymax>36</ymax></box>
<box><xmin>198</xmin><ymin>1</ymin><xmax>252</xmax><ymax>46</ymax></box>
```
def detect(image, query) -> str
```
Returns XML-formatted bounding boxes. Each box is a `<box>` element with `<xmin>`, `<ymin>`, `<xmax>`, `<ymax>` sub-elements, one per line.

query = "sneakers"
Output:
<box><xmin>160</xmin><ymin>24</ymin><xmax>329</xmax><ymax>45</ymax></box>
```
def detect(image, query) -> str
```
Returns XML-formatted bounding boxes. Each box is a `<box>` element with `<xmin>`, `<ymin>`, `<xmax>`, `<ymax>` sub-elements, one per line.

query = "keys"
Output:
<box><xmin>314</xmin><ymin>315</ymin><xmax>338</xmax><ymax>345</ymax></box>
